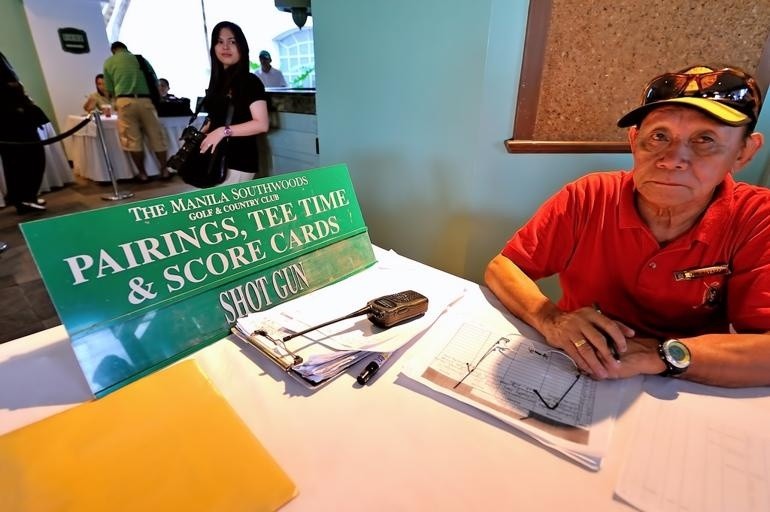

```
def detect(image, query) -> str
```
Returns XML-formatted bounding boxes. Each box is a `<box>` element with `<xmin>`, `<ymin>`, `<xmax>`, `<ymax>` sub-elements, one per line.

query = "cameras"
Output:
<box><xmin>166</xmin><ymin>125</ymin><xmax>208</xmax><ymax>170</ymax></box>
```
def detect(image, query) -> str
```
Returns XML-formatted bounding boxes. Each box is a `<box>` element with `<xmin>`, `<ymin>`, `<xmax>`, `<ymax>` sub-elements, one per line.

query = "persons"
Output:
<box><xmin>253</xmin><ymin>51</ymin><xmax>287</xmax><ymax>88</ymax></box>
<box><xmin>102</xmin><ymin>41</ymin><xmax>174</xmax><ymax>183</ymax></box>
<box><xmin>85</xmin><ymin>72</ymin><xmax>116</xmax><ymax>114</ymax></box>
<box><xmin>199</xmin><ymin>21</ymin><xmax>270</xmax><ymax>186</ymax></box>
<box><xmin>159</xmin><ymin>78</ymin><xmax>178</xmax><ymax>102</ymax></box>
<box><xmin>0</xmin><ymin>52</ymin><xmax>51</xmax><ymax>212</ymax></box>
<box><xmin>483</xmin><ymin>63</ymin><xmax>770</xmax><ymax>388</ymax></box>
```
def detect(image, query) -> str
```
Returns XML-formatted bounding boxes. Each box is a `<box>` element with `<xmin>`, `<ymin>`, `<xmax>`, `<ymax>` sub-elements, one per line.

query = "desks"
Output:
<box><xmin>64</xmin><ymin>115</ymin><xmax>195</xmax><ymax>185</ymax></box>
<box><xmin>0</xmin><ymin>242</ymin><xmax>769</xmax><ymax>511</ymax></box>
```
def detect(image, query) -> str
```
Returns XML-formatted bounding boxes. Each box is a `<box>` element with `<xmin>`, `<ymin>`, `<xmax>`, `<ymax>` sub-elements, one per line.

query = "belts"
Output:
<box><xmin>118</xmin><ymin>94</ymin><xmax>150</xmax><ymax>98</ymax></box>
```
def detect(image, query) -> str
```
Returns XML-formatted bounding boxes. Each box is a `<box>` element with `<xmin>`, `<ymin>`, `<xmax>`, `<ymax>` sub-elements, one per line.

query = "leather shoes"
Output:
<box><xmin>37</xmin><ymin>198</ymin><xmax>45</xmax><ymax>205</ymax></box>
<box><xmin>22</xmin><ymin>201</ymin><xmax>46</xmax><ymax>211</ymax></box>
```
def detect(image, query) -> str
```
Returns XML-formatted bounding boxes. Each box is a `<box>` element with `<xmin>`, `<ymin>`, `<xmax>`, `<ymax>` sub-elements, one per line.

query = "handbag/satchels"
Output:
<box><xmin>135</xmin><ymin>54</ymin><xmax>161</xmax><ymax>107</ymax></box>
<box><xmin>176</xmin><ymin>96</ymin><xmax>235</xmax><ymax>188</ymax></box>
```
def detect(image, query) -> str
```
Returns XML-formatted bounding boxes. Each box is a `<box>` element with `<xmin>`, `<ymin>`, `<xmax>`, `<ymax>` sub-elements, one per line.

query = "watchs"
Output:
<box><xmin>224</xmin><ymin>125</ymin><xmax>232</xmax><ymax>137</ymax></box>
<box><xmin>656</xmin><ymin>337</ymin><xmax>693</xmax><ymax>378</ymax></box>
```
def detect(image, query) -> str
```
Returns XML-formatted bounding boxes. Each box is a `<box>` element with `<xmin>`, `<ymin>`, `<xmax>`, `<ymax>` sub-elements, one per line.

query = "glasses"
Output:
<box><xmin>466</xmin><ymin>333</ymin><xmax>582</xmax><ymax>410</ymax></box>
<box><xmin>639</xmin><ymin>70</ymin><xmax>760</xmax><ymax>120</ymax></box>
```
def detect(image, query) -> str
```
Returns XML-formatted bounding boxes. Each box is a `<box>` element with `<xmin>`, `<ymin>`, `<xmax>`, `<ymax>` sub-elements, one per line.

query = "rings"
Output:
<box><xmin>575</xmin><ymin>337</ymin><xmax>586</xmax><ymax>348</ymax></box>
<box><xmin>208</xmin><ymin>144</ymin><xmax>211</xmax><ymax>147</ymax></box>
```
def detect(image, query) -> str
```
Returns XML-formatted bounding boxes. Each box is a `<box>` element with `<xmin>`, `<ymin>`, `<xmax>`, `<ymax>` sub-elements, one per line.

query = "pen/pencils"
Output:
<box><xmin>357</xmin><ymin>352</ymin><xmax>393</xmax><ymax>386</ymax></box>
<box><xmin>593</xmin><ymin>303</ymin><xmax>621</xmax><ymax>364</ymax></box>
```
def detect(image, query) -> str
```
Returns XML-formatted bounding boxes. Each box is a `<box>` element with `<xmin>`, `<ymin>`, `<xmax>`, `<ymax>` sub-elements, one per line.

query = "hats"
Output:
<box><xmin>617</xmin><ymin>62</ymin><xmax>762</xmax><ymax>127</ymax></box>
